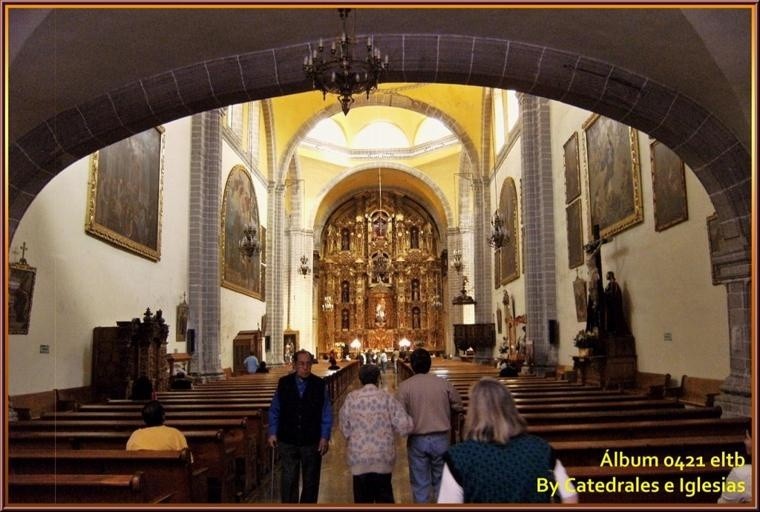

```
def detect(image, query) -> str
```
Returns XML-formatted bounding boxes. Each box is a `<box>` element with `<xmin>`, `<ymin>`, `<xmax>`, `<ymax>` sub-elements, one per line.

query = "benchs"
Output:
<box><xmin>399</xmin><ymin>357</ymin><xmax>751</xmax><ymax>503</ymax></box>
<box><xmin>7</xmin><ymin>354</ymin><xmax>360</xmax><ymax>510</ymax></box>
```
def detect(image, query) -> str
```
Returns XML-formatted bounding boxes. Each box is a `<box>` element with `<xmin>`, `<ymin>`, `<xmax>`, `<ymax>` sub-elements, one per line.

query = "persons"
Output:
<box><xmin>329</xmin><ymin>356</ymin><xmax>332</xmax><ymax>362</ymax></box>
<box><xmin>172</xmin><ymin>371</ymin><xmax>194</xmax><ymax>390</ymax></box>
<box><xmin>437</xmin><ymin>377</ymin><xmax>579</xmax><ymax>503</ymax></box>
<box><xmin>356</xmin><ymin>345</ymin><xmax>412</xmax><ymax>374</ymax></box>
<box><xmin>125</xmin><ymin>401</ymin><xmax>195</xmax><ymax>464</ymax></box>
<box><xmin>717</xmin><ymin>420</ymin><xmax>754</xmax><ymax>503</ymax></box>
<box><xmin>131</xmin><ymin>375</ymin><xmax>156</xmax><ymax>400</ymax></box>
<box><xmin>267</xmin><ymin>350</ymin><xmax>333</xmax><ymax>505</ymax></box>
<box><xmin>601</xmin><ymin>271</ymin><xmax>625</xmax><ymax>331</ymax></box>
<box><xmin>499</xmin><ymin>360</ymin><xmax>518</xmax><ymax>376</ymax></box>
<box><xmin>328</xmin><ymin>359</ymin><xmax>340</xmax><ymax>370</ymax></box>
<box><xmin>244</xmin><ymin>351</ymin><xmax>260</xmax><ymax>374</ymax></box>
<box><xmin>392</xmin><ymin>350</ymin><xmax>452</xmax><ymax>502</ymax></box>
<box><xmin>256</xmin><ymin>362</ymin><xmax>268</xmax><ymax>372</ymax></box>
<box><xmin>338</xmin><ymin>364</ymin><xmax>411</xmax><ymax>503</ymax></box>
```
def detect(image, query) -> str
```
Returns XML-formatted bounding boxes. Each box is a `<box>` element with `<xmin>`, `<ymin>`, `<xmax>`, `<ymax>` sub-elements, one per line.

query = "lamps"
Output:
<box><xmin>296</xmin><ymin>179</ymin><xmax>311</xmax><ymax>280</ymax></box>
<box><xmin>451</xmin><ymin>276</ymin><xmax>478</xmax><ymax>305</ymax></box>
<box><xmin>367</xmin><ymin>149</ymin><xmax>392</xmax><ymax>253</ymax></box>
<box><xmin>239</xmin><ymin>106</ymin><xmax>264</xmax><ymax>263</ymax></box>
<box><xmin>303</xmin><ymin>8</ymin><xmax>392</xmax><ymax>115</ymax></box>
<box><xmin>450</xmin><ymin>173</ymin><xmax>466</xmax><ymax>275</ymax></box>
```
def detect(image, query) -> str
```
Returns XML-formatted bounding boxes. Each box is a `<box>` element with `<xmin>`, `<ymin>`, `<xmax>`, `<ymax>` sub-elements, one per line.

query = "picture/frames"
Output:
<box><xmin>84</xmin><ymin>125</ymin><xmax>167</xmax><ymax>264</ymax></box>
<box><xmin>172</xmin><ymin>303</ymin><xmax>189</xmax><ymax>342</ymax></box>
<box><xmin>218</xmin><ymin>162</ymin><xmax>264</xmax><ymax>300</ymax></box>
<box><xmin>8</xmin><ymin>263</ymin><xmax>38</xmax><ymax>337</ymax></box>
<box><xmin>495</xmin><ymin>112</ymin><xmax>690</xmax><ymax>338</ymax></box>
<box><xmin>282</xmin><ymin>329</ymin><xmax>299</xmax><ymax>362</ymax></box>
